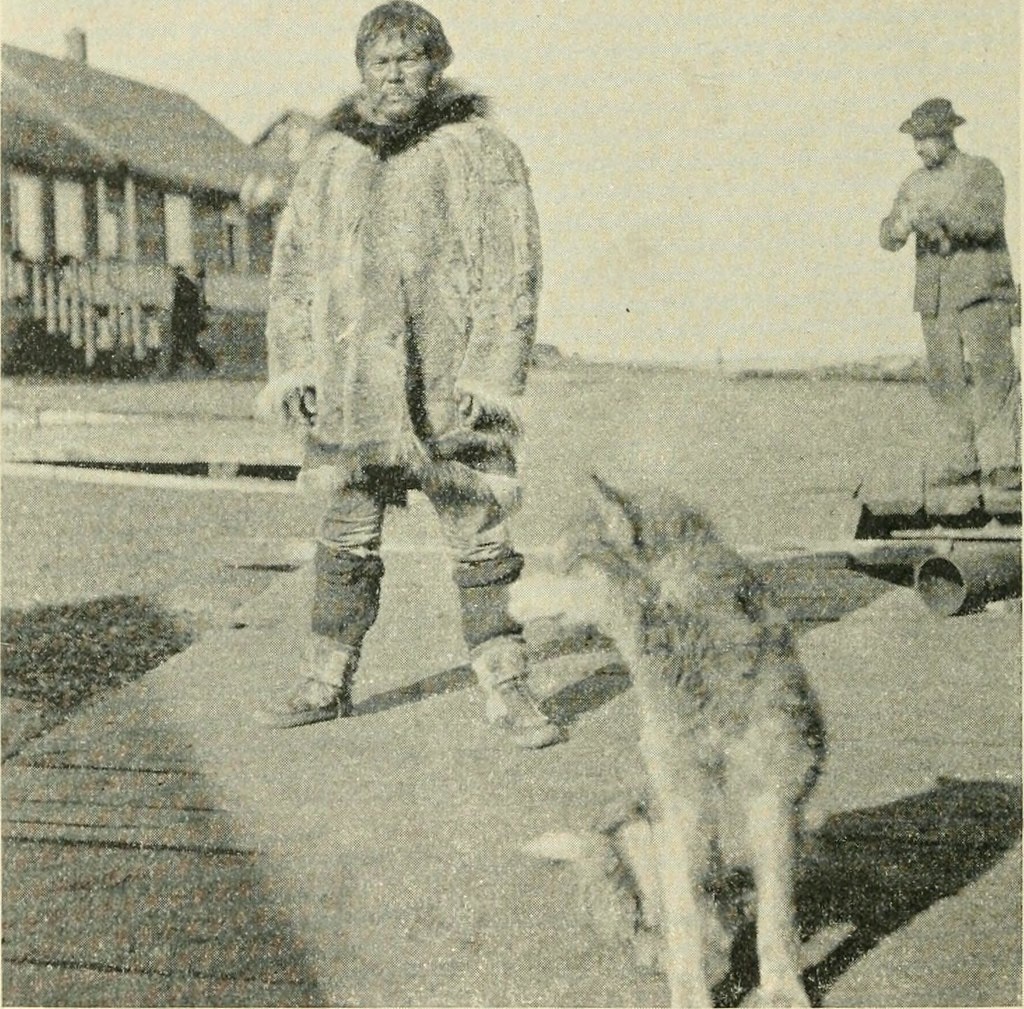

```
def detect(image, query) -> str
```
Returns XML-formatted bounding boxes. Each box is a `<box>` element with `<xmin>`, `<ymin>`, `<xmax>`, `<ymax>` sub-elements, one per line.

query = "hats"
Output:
<box><xmin>898</xmin><ymin>97</ymin><xmax>968</xmax><ymax>135</ymax></box>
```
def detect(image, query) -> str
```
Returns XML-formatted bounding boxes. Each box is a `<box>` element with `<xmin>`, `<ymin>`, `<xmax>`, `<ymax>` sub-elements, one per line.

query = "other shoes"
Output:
<box><xmin>992</xmin><ymin>465</ymin><xmax>1022</xmax><ymax>491</ymax></box>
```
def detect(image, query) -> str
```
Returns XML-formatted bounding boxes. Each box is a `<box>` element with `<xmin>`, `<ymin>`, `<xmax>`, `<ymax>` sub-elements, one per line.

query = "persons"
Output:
<box><xmin>157</xmin><ymin>264</ymin><xmax>216</xmax><ymax>378</ymax></box>
<box><xmin>251</xmin><ymin>0</ymin><xmax>559</xmax><ymax>752</ymax></box>
<box><xmin>878</xmin><ymin>99</ymin><xmax>1022</xmax><ymax>488</ymax></box>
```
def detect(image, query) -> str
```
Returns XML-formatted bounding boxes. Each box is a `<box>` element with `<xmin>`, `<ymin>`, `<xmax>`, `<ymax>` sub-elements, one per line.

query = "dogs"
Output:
<box><xmin>502</xmin><ymin>470</ymin><xmax>829</xmax><ymax>1008</ymax></box>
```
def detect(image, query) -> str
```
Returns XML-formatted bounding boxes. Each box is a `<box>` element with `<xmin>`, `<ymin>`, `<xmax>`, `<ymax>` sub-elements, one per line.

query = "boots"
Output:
<box><xmin>252</xmin><ymin>634</ymin><xmax>362</xmax><ymax>730</ymax></box>
<box><xmin>467</xmin><ymin>632</ymin><xmax>562</xmax><ymax>749</ymax></box>
